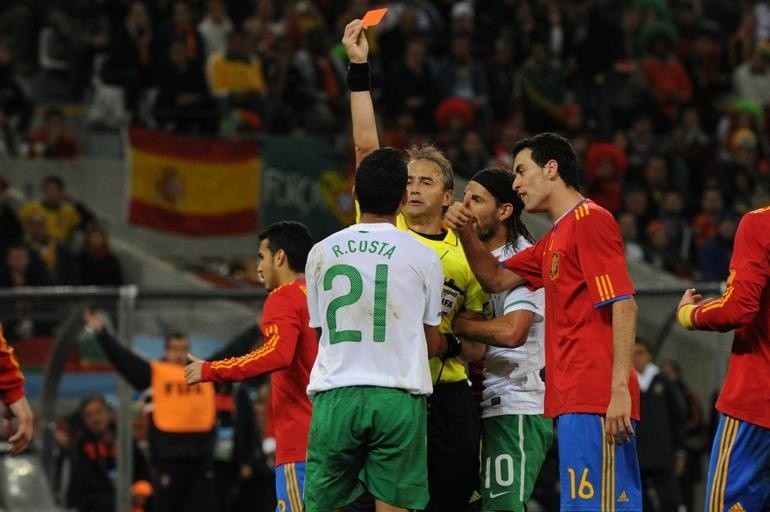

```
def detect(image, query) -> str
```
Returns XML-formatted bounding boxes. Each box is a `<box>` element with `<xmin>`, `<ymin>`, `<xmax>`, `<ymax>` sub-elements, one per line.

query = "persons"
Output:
<box><xmin>0</xmin><ymin>246</ymin><xmax>50</xmax><ymax>334</ymax></box>
<box><xmin>224</xmin><ymin>334</ymin><xmax>276</xmax><ymax>512</ymax></box>
<box><xmin>185</xmin><ymin>222</ymin><xmax>318</xmax><ymax>512</ymax></box>
<box><xmin>18</xmin><ymin>176</ymin><xmax>98</xmax><ymax>266</ymax></box>
<box><xmin>635</xmin><ymin>336</ymin><xmax>677</xmax><ymax>512</ymax></box>
<box><xmin>574</xmin><ymin>0</ymin><xmax>770</xmax><ymax>283</ymax></box>
<box><xmin>444</xmin><ymin>132</ymin><xmax>642</xmax><ymax>512</ymax></box>
<box><xmin>0</xmin><ymin>0</ymin><xmax>340</xmax><ymax>147</ymax></box>
<box><xmin>662</xmin><ymin>360</ymin><xmax>702</xmax><ymax>512</ymax></box>
<box><xmin>676</xmin><ymin>206</ymin><xmax>770</xmax><ymax>512</ymax></box>
<box><xmin>0</xmin><ymin>324</ymin><xmax>33</xmax><ymax>458</ymax></box>
<box><xmin>44</xmin><ymin>397</ymin><xmax>156</xmax><ymax>512</ymax></box>
<box><xmin>342</xmin><ymin>18</ymin><xmax>492</xmax><ymax>512</ymax></box>
<box><xmin>0</xmin><ymin>177</ymin><xmax>25</xmax><ymax>265</ymax></box>
<box><xmin>65</xmin><ymin>224</ymin><xmax>126</xmax><ymax>310</ymax></box>
<box><xmin>303</xmin><ymin>149</ymin><xmax>443</xmax><ymax>512</ymax></box>
<box><xmin>83</xmin><ymin>308</ymin><xmax>268</xmax><ymax>512</ymax></box>
<box><xmin>452</xmin><ymin>168</ymin><xmax>554</xmax><ymax>512</ymax></box>
